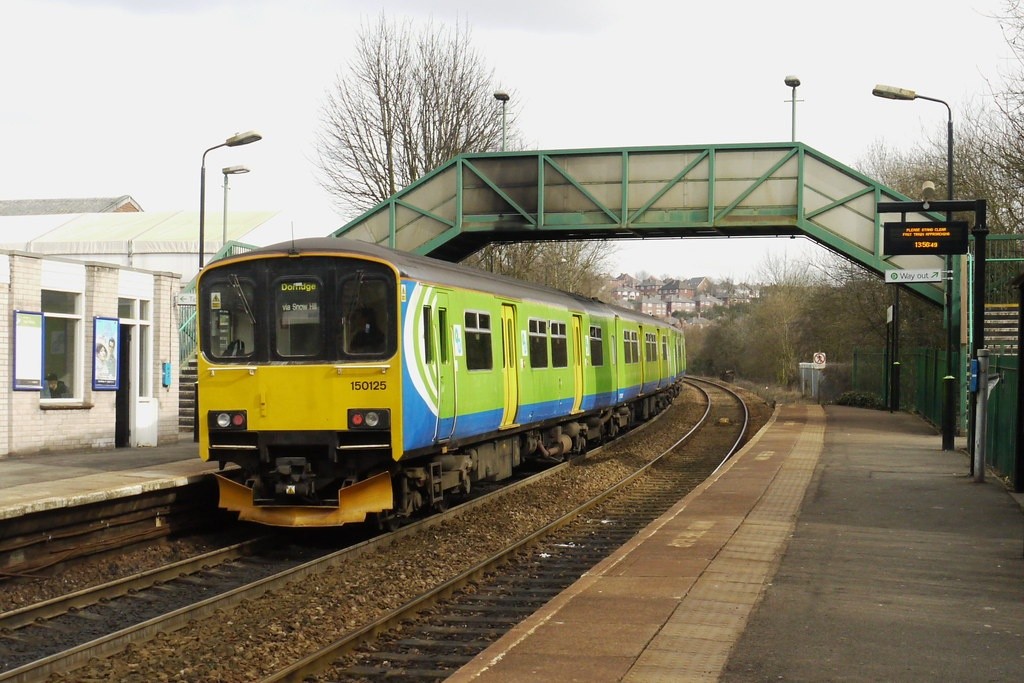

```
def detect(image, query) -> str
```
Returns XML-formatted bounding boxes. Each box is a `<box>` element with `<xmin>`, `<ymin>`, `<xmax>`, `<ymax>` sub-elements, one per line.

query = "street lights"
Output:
<box><xmin>782</xmin><ymin>77</ymin><xmax>804</xmax><ymax>142</ymax></box>
<box><xmin>492</xmin><ymin>90</ymin><xmax>515</xmax><ymax>150</ymax></box>
<box><xmin>196</xmin><ymin>133</ymin><xmax>263</xmax><ymax>443</ymax></box>
<box><xmin>872</xmin><ymin>84</ymin><xmax>959</xmax><ymax>449</ymax></box>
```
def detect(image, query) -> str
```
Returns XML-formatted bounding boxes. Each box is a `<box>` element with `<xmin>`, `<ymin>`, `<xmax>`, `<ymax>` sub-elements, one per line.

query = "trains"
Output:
<box><xmin>194</xmin><ymin>221</ymin><xmax>688</xmax><ymax>545</ymax></box>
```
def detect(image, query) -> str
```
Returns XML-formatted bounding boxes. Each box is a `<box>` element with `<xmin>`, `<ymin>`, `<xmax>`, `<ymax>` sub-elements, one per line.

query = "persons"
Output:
<box><xmin>95</xmin><ymin>338</ymin><xmax>117</xmax><ymax>381</ymax></box>
<box><xmin>44</xmin><ymin>372</ymin><xmax>73</xmax><ymax>398</ymax></box>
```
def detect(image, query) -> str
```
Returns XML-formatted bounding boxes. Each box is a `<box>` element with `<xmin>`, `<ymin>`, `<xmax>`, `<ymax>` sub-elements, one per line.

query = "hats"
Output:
<box><xmin>44</xmin><ymin>373</ymin><xmax>57</xmax><ymax>379</ymax></box>
